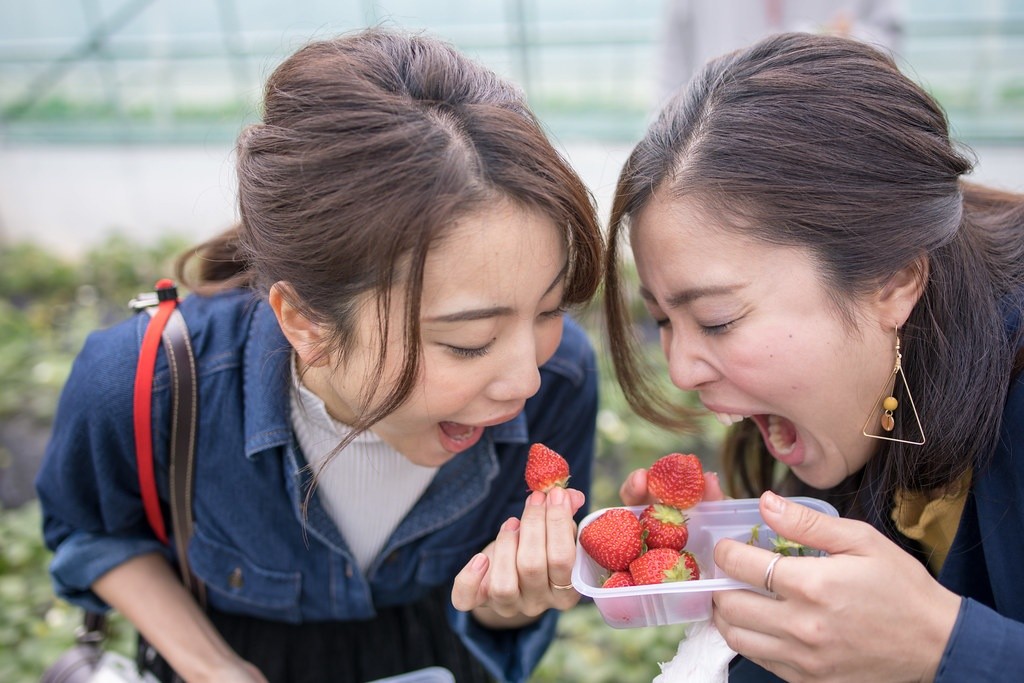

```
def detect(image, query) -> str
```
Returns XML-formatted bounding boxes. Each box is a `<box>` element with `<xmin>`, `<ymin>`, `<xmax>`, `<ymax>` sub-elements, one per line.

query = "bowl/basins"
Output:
<box><xmin>569</xmin><ymin>495</ymin><xmax>839</xmax><ymax>630</ymax></box>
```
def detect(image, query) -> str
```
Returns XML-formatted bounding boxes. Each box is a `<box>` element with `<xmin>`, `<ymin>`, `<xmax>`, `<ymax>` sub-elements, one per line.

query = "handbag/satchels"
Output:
<box><xmin>37</xmin><ymin>640</ymin><xmax>161</xmax><ymax>683</ymax></box>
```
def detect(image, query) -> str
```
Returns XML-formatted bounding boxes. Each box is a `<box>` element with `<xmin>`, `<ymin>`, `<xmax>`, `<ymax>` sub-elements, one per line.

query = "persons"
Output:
<box><xmin>602</xmin><ymin>28</ymin><xmax>1023</xmax><ymax>683</ymax></box>
<box><xmin>38</xmin><ymin>35</ymin><xmax>609</xmax><ymax>681</ymax></box>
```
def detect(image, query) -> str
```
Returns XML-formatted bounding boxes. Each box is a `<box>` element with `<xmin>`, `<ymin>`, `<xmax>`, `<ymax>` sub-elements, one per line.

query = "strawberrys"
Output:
<box><xmin>578</xmin><ymin>509</ymin><xmax>648</xmax><ymax>571</ymax></box>
<box><xmin>639</xmin><ymin>502</ymin><xmax>688</xmax><ymax>553</ymax></box>
<box><xmin>646</xmin><ymin>453</ymin><xmax>705</xmax><ymax>509</ymax></box>
<box><xmin>524</xmin><ymin>442</ymin><xmax>569</xmax><ymax>493</ymax></box>
<box><xmin>598</xmin><ymin>568</ymin><xmax>639</xmax><ymax>623</ymax></box>
<box><xmin>680</xmin><ymin>550</ymin><xmax>699</xmax><ymax>581</ymax></box>
<box><xmin>629</xmin><ymin>546</ymin><xmax>692</xmax><ymax>585</ymax></box>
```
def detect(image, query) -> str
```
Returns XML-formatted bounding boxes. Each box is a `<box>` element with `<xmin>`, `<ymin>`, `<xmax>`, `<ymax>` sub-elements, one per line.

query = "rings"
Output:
<box><xmin>548</xmin><ymin>578</ymin><xmax>575</xmax><ymax>592</ymax></box>
<box><xmin>764</xmin><ymin>552</ymin><xmax>783</xmax><ymax>593</ymax></box>
